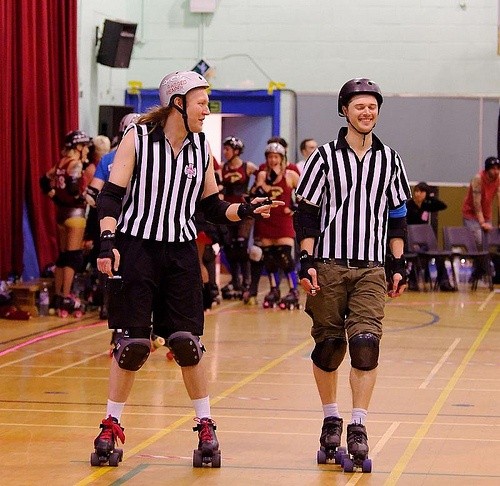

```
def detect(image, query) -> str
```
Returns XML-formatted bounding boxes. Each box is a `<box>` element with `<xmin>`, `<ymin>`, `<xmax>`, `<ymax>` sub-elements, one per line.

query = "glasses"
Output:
<box><xmin>490</xmin><ymin>159</ymin><xmax>499</xmax><ymax>165</ymax></box>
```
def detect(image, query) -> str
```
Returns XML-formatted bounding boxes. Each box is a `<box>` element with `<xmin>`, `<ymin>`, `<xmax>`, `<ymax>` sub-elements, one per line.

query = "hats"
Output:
<box><xmin>485</xmin><ymin>157</ymin><xmax>500</xmax><ymax>170</ymax></box>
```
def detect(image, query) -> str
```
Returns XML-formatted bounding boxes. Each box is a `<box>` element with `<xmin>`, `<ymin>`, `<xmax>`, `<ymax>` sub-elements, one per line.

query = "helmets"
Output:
<box><xmin>159</xmin><ymin>71</ymin><xmax>210</xmax><ymax>107</ymax></box>
<box><xmin>338</xmin><ymin>79</ymin><xmax>383</xmax><ymax>117</ymax></box>
<box><xmin>119</xmin><ymin>113</ymin><xmax>142</xmax><ymax>130</ymax></box>
<box><xmin>264</xmin><ymin>143</ymin><xmax>286</xmax><ymax>158</ymax></box>
<box><xmin>223</xmin><ymin>136</ymin><xmax>244</xmax><ymax>149</ymax></box>
<box><xmin>66</xmin><ymin>131</ymin><xmax>91</xmax><ymax>147</ymax></box>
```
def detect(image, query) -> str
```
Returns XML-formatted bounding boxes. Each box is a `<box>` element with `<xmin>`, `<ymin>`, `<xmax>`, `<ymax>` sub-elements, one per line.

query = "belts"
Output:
<box><xmin>316</xmin><ymin>257</ymin><xmax>382</xmax><ymax>268</ymax></box>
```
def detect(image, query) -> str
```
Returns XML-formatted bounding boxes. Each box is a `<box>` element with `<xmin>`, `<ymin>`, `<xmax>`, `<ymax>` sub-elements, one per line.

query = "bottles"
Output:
<box><xmin>39</xmin><ymin>282</ymin><xmax>50</xmax><ymax>317</ymax></box>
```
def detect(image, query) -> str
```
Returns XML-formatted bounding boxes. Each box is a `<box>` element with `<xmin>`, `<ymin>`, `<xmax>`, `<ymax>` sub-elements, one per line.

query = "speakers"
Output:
<box><xmin>97</xmin><ymin>105</ymin><xmax>134</xmax><ymax>146</ymax></box>
<box><xmin>95</xmin><ymin>18</ymin><xmax>137</xmax><ymax>68</ymax></box>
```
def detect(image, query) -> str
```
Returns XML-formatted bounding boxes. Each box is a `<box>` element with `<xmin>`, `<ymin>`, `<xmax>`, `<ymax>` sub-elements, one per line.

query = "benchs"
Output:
<box><xmin>8</xmin><ymin>278</ymin><xmax>53</xmax><ymax>314</ymax></box>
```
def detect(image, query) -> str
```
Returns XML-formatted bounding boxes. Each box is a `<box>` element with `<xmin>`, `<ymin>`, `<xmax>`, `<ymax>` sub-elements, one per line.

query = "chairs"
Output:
<box><xmin>404</xmin><ymin>224</ymin><xmax>500</xmax><ymax>292</ymax></box>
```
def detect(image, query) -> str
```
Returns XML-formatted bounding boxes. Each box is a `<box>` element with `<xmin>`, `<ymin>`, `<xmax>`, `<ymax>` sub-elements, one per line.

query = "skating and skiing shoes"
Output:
<box><xmin>278</xmin><ymin>289</ymin><xmax>304</xmax><ymax>310</ymax></box>
<box><xmin>220</xmin><ymin>281</ymin><xmax>246</xmax><ymax>300</ymax></box>
<box><xmin>243</xmin><ymin>287</ymin><xmax>257</xmax><ymax>304</ymax></box>
<box><xmin>49</xmin><ymin>294</ymin><xmax>62</xmax><ymax>314</ymax></box>
<box><xmin>341</xmin><ymin>424</ymin><xmax>372</xmax><ymax>473</ymax></box>
<box><xmin>193</xmin><ymin>417</ymin><xmax>222</xmax><ymax>468</ymax></box>
<box><xmin>317</xmin><ymin>416</ymin><xmax>346</xmax><ymax>464</ymax></box>
<box><xmin>264</xmin><ymin>287</ymin><xmax>281</xmax><ymax>308</ymax></box>
<box><xmin>91</xmin><ymin>414</ymin><xmax>125</xmax><ymax>467</ymax></box>
<box><xmin>57</xmin><ymin>297</ymin><xmax>86</xmax><ymax>319</ymax></box>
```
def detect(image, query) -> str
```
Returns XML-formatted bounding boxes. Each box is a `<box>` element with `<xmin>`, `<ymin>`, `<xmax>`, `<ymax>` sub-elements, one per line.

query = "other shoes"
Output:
<box><xmin>99</xmin><ymin>299</ymin><xmax>108</xmax><ymax>319</ymax></box>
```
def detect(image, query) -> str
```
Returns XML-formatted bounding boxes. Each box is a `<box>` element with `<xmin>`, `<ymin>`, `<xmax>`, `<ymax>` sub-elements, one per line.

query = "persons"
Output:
<box><xmin>461</xmin><ymin>157</ymin><xmax>500</xmax><ymax>277</ymax></box>
<box><xmin>292</xmin><ymin>78</ymin><xmax>412</xmax><ymax>474</ymax></box>
<box><xmin>40</xmin><ymin>130</ymin><xmax>448</xmax><ymax>318</ymax></box>
<box><xmin>89</xmin><ymin>70</ymin><xmax>286</xmax><ymax>468</ymax></box>
